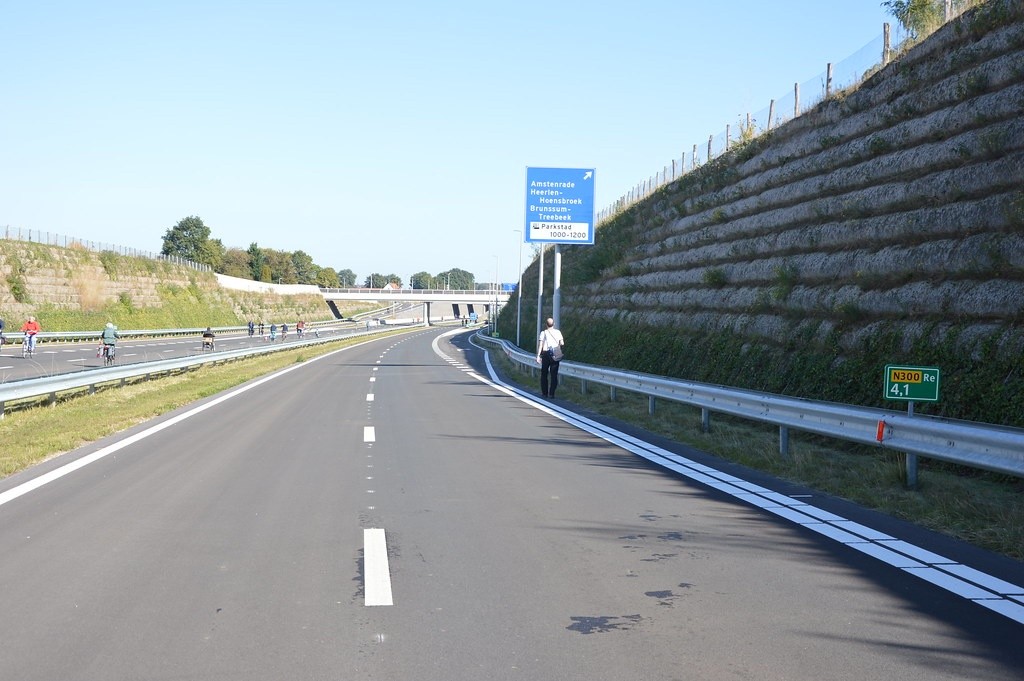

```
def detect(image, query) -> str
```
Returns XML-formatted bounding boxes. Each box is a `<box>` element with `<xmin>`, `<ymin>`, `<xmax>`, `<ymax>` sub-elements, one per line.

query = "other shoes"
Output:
<box><xmin>541</xmin><ymin>393</ymin><xmax>548</xmax><ymax>398</ymax></box>
<box><xmin>549</xmin><ymin>395</ymin><xmax>555</xmax><ymax>399</ymax></box>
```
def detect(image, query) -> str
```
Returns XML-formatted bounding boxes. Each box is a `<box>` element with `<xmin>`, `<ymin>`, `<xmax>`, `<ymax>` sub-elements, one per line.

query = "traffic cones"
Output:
<box><xmin>97</xmin><ymin>340</ymin><xmax>104</xmax><ymax>359</ymax></box>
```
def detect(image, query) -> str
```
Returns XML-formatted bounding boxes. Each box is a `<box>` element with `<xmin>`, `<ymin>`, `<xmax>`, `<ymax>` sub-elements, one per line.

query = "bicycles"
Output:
<box><xmin>103</xmin><ymin>343</ymin><xmax>115</xmax><ymax>366</ymax></box>
<box><xmin>248</xmin><ymin>326</ymin><xmax>302</xmax><ymax>343</ymax></box>
<box><xmin>21</xmin><ymin>329</ymin><xmax>35</xmax><ymax>359</ymax></box>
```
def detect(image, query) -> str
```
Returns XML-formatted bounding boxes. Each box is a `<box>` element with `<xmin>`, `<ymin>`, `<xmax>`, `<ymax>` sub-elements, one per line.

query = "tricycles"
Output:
<box><xmin>201</xmin><ymin>338</ymin><xmax>215</xmax><ymax>352</ymax></box>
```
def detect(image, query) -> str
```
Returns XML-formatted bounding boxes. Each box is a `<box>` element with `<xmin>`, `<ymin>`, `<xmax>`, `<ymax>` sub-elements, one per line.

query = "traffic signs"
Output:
<box><xmin>523</xmin><ymin>166</ymin><xmax>597</xmax><ymax>245</ymax></box>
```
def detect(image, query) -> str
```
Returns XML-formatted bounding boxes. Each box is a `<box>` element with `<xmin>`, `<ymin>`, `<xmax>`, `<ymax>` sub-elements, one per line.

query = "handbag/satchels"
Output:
<box><xmin>543</xmin><ymin>330</ymin><xmax>563</xmax><ymax>361</ymax></box>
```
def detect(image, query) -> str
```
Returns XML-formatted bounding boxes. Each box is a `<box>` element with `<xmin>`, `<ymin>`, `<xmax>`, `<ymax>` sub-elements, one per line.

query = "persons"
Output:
<box><xmin>537</xmin><ymin>318</ymin><xmax>564</xmax><ymax>398</ymax></box>
<box><xmin>20</xmin><ymin>317</ymin><xmax>38</xmax><ymax>352</ymax></box>
<box><xmin>202</xmin><ymin>327</ymin><xmax>216</xmax><ymax>348</ymax></box>
<box><xmin>248</xmin><ymin>318</ymin><xmax>322</xmax><ymax>341</ymax></box>
<box><xmin>461</xmin><ymin>313</ymin><xmax>479</xmax><ymax>328</ymax></box>
<box><xmin>366</xmin><ymin>322</ymin><xmax>370</xmax><ymax>331</ymax></box>
<box><xmin>0</xmin><ymin>319</ymin><xmax>4</xmax><ymax>339</ymax></box>
<box><xmin>101</xmin><ymin>322</ymin><xmax>120</xmax><ymax>363</ymax></box>
